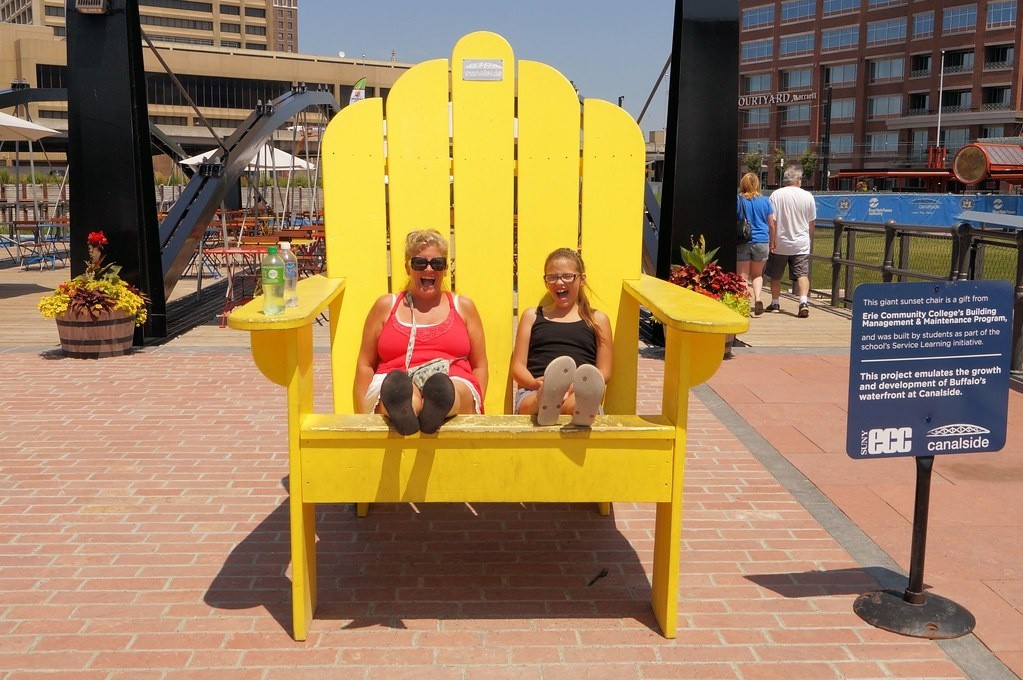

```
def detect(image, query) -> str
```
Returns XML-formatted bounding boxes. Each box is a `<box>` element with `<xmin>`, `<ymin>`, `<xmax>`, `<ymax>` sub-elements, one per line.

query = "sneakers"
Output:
<box><xmin>765</xmin><ymin>304</ymin><xmax>779</xmax><ymax>313</ymax></box>
<box><xmin>798</xmin><ymin>302</ymin><xmax>809</xmax><ymax>317</ymax></box>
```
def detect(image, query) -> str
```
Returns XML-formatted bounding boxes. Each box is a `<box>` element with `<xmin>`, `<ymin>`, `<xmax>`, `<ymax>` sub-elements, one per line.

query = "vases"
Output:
<box><xmin>723</xmin><ymin>332</ymin><xmax>733</xmax><ymax>359</ymax></box>
<box><xmin>55</xmin><ymin>306</ymin><xmax>134</xmax><ymax>358</ymax></box>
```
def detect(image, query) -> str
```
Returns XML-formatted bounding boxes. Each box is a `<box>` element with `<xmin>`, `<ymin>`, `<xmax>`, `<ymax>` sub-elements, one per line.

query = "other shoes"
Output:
<box><xmin>573</xmin><ymin>363</ymin><xmax>605</xmax><ymax>426</ymax></box>
<box><xmin>755</xmin><ymin>300</ymin><xmax>763</xmax><ymax>315</ymax></box>
<box><xmin>537</xmin><ymin>356</ymin><xmax>577</xmax><ymax>426</ymax></box>
<box><xmin>417</xmin><ymin>373</ymin><xmax>455</xmax><ymax>434</ymax></box>
<box><xmin>380</xmin><ymin>370</ymin><xmax>419</xmax><ymax>435</ymax></box>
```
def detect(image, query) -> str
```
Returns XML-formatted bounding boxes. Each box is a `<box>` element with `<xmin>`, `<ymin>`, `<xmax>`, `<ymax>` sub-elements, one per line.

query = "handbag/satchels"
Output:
<box><xmin>409</xmin><ymin>357</ymin><xmax>450</xmax><ymax>397</ymax></box>
<box><xmin>735</xmin><ymin>195</ymin><xmax>752</xmax><ymax>244</ymax></box>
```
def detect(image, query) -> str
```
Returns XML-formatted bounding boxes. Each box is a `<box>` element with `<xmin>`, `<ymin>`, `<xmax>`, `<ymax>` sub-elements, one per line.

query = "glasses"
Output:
<box><xmin>411</xmin><ymin>257</ymin><xmax>446</xmax><ymax>271</ymax></box>
<box><xmin>544</xmin><ymin>273</ymin><xmax>580</xmax><ymax>284</ymax></box>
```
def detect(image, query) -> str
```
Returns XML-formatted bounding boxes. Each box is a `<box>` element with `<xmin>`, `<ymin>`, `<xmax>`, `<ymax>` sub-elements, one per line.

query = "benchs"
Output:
<box><xmin>159</xmin><ymin>210</ymin><xmax>326</xmax><ymax>272</ymax></box>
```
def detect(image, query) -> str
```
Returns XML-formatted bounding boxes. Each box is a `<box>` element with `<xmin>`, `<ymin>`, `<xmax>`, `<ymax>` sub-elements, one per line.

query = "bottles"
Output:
<box><xmin>262</xmin><ymin>246</ymin><xmax>286</xmax><ymax>316</ymax></box>
<box><xmin>276</xmin><ymin>242</ymin><xmax>298</xmax><ymax>307</ymax></box>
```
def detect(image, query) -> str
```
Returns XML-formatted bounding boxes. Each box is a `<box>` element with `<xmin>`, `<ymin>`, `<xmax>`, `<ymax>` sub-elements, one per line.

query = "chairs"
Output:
<box><xmin>229</xmin><ymin>30</ymin><xmax>750</xmax><ymax>638</ymax></box>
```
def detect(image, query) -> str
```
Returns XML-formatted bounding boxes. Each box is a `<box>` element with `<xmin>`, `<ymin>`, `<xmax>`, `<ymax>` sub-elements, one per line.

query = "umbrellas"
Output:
<box><xmin>179</xmin><ymin>138</ymin><xmax>317</xmax><ymax>171</ymax></box>
<box><xmin>0</xmin><ymin>110</ymin><xmax>62</xmax><ymax>142</ymax></box>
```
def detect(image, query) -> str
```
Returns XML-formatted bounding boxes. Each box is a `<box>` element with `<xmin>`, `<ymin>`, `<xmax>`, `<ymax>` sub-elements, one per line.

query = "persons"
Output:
<box><xmin>766</xmin><ymin>168</ymin><xmax>816</xmax><ymax>318</ymax></box>
<box><xmin>857</xmin><ymin>179</ymin><xmax>867</xmax><ymax>192</ymax></box>
<box><xmin>737</xmin><ymin>173</ymin><xmax>776</xmax><ymax>315</ymax></box>
<box><xmin>252</xmin><ymin>194</ymin><xmax>264</xmax><ymax>209</ymax></box>
<box><xmin>510</xmin><ymin>248</ymin><xmax>613</xmax><ymax>426</ymax></box>
<box><xmin>353</xmin><ymin>228</ymin><xmax>489</xmax><ymax>435</ymax></box>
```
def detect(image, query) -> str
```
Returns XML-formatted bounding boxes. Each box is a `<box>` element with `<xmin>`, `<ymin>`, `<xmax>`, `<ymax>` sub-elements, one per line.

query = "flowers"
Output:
<box><xmin>668</xmin><ymin>233</ymin><xmax>751</xmax><ymax>318</ymax></box>
<box><xmin>38</xmin><ymin>232</ymin><xmax>152</xmax><ymax>329</ymax></box>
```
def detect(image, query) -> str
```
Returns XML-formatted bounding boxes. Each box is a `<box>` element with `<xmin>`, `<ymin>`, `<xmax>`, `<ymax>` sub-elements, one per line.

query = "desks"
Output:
<box><xmin>205</xmin><ymin>247</ymin><xmax>270</xmax><ymax>274</ymax></box>
